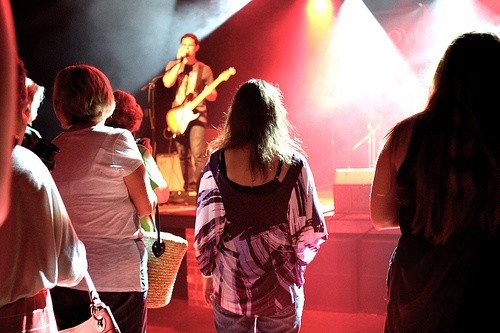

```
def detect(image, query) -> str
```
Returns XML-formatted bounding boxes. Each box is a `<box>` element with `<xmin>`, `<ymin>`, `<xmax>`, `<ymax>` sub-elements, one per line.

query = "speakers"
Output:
<box><xmin>333</xmin><ymin>168</ymin><xmax>375</xmax><ymax>214</ymax></box>
<box><xmin>157</xmin><ymin>153</ymin><xmax>185</xmax><ymax>195</ymax></box>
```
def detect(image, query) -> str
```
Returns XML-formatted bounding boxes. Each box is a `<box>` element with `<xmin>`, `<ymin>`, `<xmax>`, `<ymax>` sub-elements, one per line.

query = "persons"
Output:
<box><xmin>370</xmin><ymin>33</ymin><xmax>500</xmax><ymax>333</ymax></box>
<box><xmin>163</xmin><ymin>33</ymin><xmax>217</xmax><ymax>196</ymax></box>
<box><xmin>21</xmin><ymin>77</ymin><xmax>45</xmax><ymax>156</ymax></box>
<box><xmin>192</xmin><ymin>79</ymin><xmax>329</xmax><ymax>333</ymax></box>
<box><xmin>51</xmin><ymin>64</ymin><xmax>166</xmax><ymax>333</ymax></box>
<box><xmin>0</xmin><ymin>55</ymin><xmax>89</xmax><ymax>333</ymax></box>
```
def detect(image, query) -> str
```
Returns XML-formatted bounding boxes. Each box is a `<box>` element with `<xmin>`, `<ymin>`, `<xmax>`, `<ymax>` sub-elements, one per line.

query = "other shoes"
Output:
<box><xmin>182</xmin><ymin>189</ymin><xmax>197</xmax><ymax>206</ymax></box>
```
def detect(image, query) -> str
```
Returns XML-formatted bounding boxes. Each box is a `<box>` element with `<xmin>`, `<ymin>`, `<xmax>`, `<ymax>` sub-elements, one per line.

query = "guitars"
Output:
<box><xmin>166</xmin><ymin>66</ymin><xmax>236</xmax><ymax>136</ymax></box>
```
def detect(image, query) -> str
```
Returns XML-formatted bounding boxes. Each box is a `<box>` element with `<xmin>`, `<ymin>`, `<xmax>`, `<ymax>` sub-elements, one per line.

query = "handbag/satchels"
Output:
<box><xmin>58</xmin><ymin>272</ymin><xmax>122</xmax><ymax>333</ymax></box>
<box><xmin>140</xmin><ymin>203</ymin><xmax>187</xmax><ymax>308</ymax></box>
